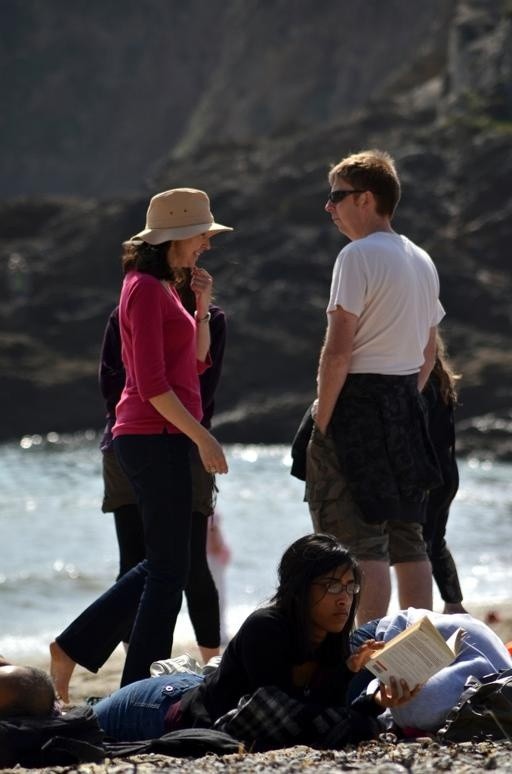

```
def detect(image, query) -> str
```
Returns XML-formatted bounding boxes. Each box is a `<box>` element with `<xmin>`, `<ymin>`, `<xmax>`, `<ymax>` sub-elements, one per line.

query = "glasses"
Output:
<box><xmin>312</xmin><ymin>580</ymin><xmax>360</xmax><ymax>594</ymax></box>
<box><xmin>326</xmin><ymin>189</ymin><xmax>363</xmax><ymax>202</ymax></box>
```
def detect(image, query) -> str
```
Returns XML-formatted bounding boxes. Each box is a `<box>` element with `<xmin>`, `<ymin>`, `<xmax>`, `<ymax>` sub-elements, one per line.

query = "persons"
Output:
<box><xmin>92</xmin><ymin>531</ymin><xmax>427</xmax><ymax>752</ymax></box>
<box><xmin>422</xmin><ymin>336</ymin><xmax>469</xmax><ymax>618</ymax></box>
<box><xmin>48</xmin><ymin>187</ymin><xmax>236</xmax><ymax>707</ymax></box>
<box><xmin>95</xmin><ymin>263</ymin><xmax>228</xmax><ymax>667</ymax></box>
<box><xmin>302</xmin><ymin>145</ymin><xmax>448</xmax><ymax>631</ymax></box>
<box><xmin>0</xmin><ymin>653</ymin><xmax>59</xmax><ymax>721</ymax></box>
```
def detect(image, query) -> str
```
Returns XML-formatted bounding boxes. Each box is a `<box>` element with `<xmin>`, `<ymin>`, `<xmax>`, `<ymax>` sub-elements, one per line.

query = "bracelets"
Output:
<box><xmin>193</xmin><ymin>310</ymin><xmax>213</xmax><ymax>325</ymax></box>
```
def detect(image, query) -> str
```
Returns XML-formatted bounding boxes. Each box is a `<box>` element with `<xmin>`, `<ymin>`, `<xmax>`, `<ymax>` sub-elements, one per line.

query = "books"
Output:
<box><xmin>363</xmin><ymin>616</ymin><xmax>478</xmax><ymax>699</ymax></box>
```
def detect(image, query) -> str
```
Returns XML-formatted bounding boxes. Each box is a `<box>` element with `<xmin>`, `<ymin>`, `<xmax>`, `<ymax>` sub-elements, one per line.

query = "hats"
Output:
<box><xmin>130</xmin><ymin>188</ymin><xmax>234</xmax><ymax>245</ymax></box>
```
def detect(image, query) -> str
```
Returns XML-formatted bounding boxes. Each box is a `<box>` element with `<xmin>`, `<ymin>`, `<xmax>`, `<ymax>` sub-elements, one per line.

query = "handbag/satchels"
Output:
<box><xmin>436</xmin><ymin>668</ymin><xmax>512</xmax><ymax>741</ymax></box>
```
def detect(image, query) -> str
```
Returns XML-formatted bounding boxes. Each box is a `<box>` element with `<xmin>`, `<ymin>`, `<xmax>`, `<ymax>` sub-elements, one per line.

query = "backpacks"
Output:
<box><xmin>0</xmin><ymin>704</ymin><xmax>104</xmax><ymax>764</ymax></box>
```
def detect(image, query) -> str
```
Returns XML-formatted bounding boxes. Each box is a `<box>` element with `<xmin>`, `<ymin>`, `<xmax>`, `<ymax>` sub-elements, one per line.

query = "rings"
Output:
<box><xmin>211</xmin><ymin>466</ymin><xmax>219</xmax><ymax>474</ymax></box>
